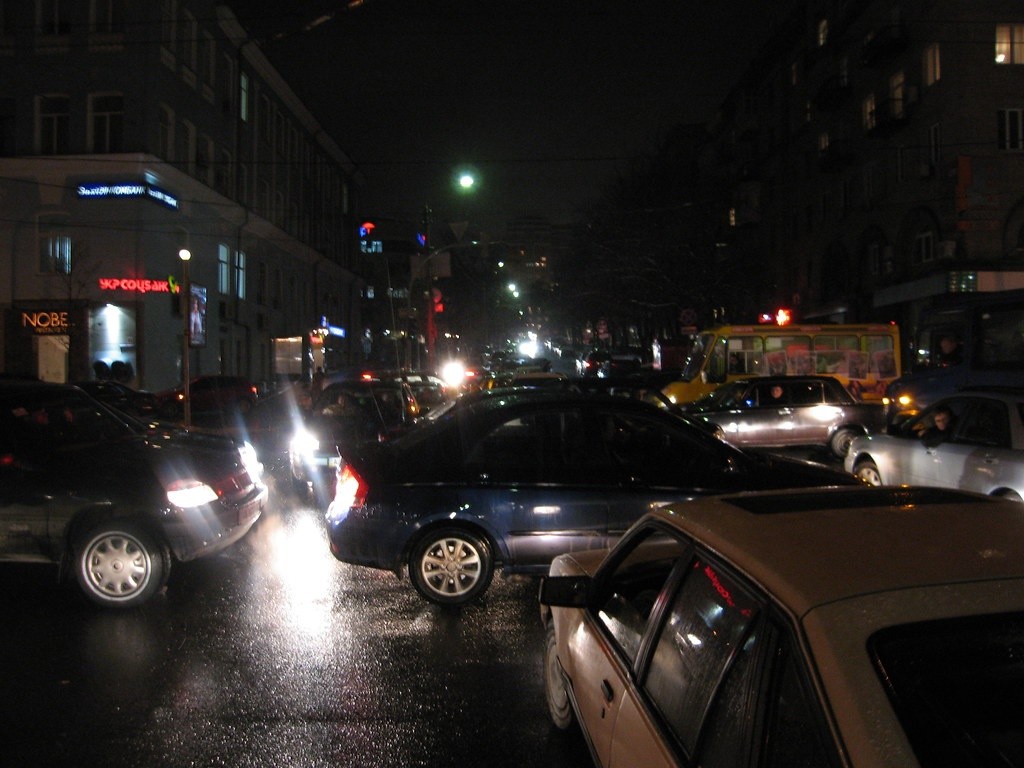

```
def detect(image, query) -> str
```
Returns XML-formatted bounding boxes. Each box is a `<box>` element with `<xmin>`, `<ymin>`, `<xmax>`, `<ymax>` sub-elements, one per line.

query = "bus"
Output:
<box><xmin>652</xmin><ymin>308</ymin><xmax>904</xmax><ymax>408</ymax></box>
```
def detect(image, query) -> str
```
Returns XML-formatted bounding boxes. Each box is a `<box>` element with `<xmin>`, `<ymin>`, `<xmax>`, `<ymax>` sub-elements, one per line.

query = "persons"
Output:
<box><xmin>93</xmin><ymin>361</ymin><xmax>139</xmax><ymax>401</ymax></box>
<box><xmin>940</xmin><ymin>337</ymin><xmax>963</xmax><ymax>368</ymax></box>
<box><xmin>729</xmin><ymin>355</ymin><xmax>745</xmax><ymax>373</ymax></box>
<box><xmin>490</xmin><ymin>350</ymin><xmax>518</xmax><ymax>373</ymax></box>
<box><xmin>917</xmin><ymin>405</ymin><xmax>957</xmax><ymax>448</ymax></box>
<box><xmin>761</xmin><ymin>384</ymin><xmax>788</xmax><ymax>406</ymax></box>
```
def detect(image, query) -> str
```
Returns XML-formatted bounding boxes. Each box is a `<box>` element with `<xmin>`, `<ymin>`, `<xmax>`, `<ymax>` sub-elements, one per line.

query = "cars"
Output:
<box><xmin>164</xmin><ymin>370</ymin><xmax>1024</xmax><ymax>506</ymax></box>
<box><xmin>1</xmin><ymin>373</ymin><xmax>270</xmax><ymax>613</ymax></box>
<box><xmin>540</xmin><ymin>484</ymin><xmax>1024</xmax><ymax>768</ymax></box>
<box><xmin>320</xmin><ymin>376</ymin><xmax>877</xmax><ymax>610</ymax></box>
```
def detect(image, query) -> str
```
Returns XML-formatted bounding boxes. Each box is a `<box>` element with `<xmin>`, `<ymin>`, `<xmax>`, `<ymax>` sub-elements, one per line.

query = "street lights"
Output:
<box><xmin>175</xmin><ymin>217</ymin><xmax>193</xmax><ymax>430</ymax></box>
